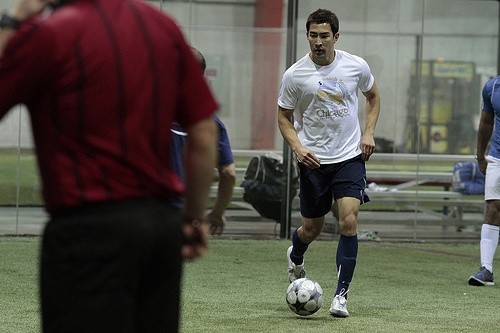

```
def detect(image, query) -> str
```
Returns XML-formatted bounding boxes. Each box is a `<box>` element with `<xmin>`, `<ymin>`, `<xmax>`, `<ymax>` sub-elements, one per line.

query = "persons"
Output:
<box><xmin>0</xmin><ymin>1</ymin><xmax>239</xmax><ymax>333</ymax></box>
<box><xmin>467</xmin><ymin>77</ymin><xmax>500</xmax><ymax>287</ymax></box>
<box><xmin>277</xmin><ymin>9</ymin><xmax>380</xmax><ymax>319</ymax></box>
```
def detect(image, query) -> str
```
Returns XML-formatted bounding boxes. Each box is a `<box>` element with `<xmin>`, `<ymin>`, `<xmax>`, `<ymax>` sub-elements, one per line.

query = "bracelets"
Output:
<box><xmin>0</xmin><ymin>12</ymin><xmax>21</xmax><ymax>32</ymax></box>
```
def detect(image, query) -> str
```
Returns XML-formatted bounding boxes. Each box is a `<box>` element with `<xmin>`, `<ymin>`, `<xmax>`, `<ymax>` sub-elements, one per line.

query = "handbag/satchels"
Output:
<box><xmin>452</xmin><ymin>159</ymin><xmax>485</xmax><ymax>193</ymax></box>
<box><xmin>239</xmin><ymin>155</ymin><xmax>300</xmax><ymax>224</ymax></box>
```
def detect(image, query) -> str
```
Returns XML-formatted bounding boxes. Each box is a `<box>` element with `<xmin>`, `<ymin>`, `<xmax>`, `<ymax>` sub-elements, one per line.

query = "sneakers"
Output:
<box><xmin>286</xmin><ymin>246</ymin><xmax>307</xmax><ymax>284</ymax></box>
<box><xmin>329</xmin><ymin>288</ymin><xmax>350</xmax><ymax>318</ymax></box>
<box><xmin>467</xmin><ymin>266</ymin><xmax>495</xmax><ymax>285</ymax></box>
<box><xmin>356</xmin><ymin>229</ymin><xmax>382</xmax><ymax>242</ymax></box>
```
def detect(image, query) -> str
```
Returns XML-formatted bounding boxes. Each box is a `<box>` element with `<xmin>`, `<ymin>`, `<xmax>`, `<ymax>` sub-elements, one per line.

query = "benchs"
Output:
<box><xmin>207</xmin><ymin>148</ymin><xmax>485</xmax><ymax>243</ymax></box>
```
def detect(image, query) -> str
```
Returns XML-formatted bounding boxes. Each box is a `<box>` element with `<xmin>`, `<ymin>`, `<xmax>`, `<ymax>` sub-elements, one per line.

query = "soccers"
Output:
<box><xmin>285</xmin><ymin>277</ymin><xmax>325</xmax><ymax>317</ymax></box>
